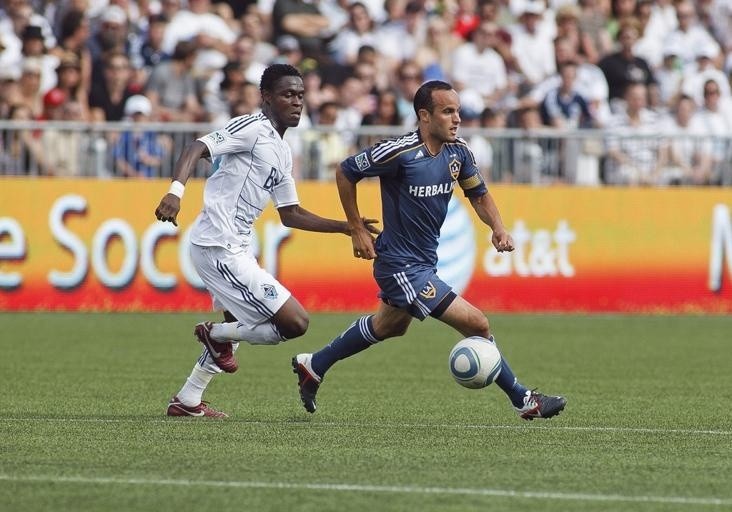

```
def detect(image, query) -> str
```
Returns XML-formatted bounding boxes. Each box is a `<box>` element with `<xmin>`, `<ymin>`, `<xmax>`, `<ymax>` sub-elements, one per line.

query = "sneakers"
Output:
<box><xmin>192</xmin><ymin>320</ymin><xmax>240</xmax><ymax>374</ymax></box>
<box><xmin>291</xmin><ymin>352</ymin><xmax>323</xmax><ymax>414</ymax></box>
<box><xmin>165</xmin><ymin>395</ymin><xmax>230</xmax><ymax>419</ymax></box>
<box><xmin>513</xmin><ymin>386</ymin><xmax>569</xmax><ymax>422</ymax></box>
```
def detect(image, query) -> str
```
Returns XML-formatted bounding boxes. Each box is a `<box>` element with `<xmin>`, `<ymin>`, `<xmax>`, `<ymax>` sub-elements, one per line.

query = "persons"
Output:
<box><xmin>3</xmin><ymin>0</ymin><xmax>731</xmax><ymax>188</ymax></box>
<box><xmin>153</xmin><ymin>64</ymin><xmax>383</xmax><ymax>420</ymax></box>
<box><xmin>291</xmin><ymin>79</ymin><xmax>568</xmax><ymax>420</ymax></box>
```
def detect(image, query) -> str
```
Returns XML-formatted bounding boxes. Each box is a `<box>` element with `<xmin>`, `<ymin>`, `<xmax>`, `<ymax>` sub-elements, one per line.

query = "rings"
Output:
<box><xmin>168</xmin><ymin>215</ymin><xmax>174</xmax><ymax>221</ymax></box>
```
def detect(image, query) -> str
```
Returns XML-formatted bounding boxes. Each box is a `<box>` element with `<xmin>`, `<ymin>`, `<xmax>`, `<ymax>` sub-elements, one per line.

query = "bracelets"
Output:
<box><xmin>168</xmin><ymin>180</ymin><xmax>185</xmax><ymax>199</ymax></box>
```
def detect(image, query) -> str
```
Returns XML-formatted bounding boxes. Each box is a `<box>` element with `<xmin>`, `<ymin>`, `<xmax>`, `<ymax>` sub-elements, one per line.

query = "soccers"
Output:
<box><xmin>449</xmin><ymin>335</ymin><xmax>502</xmax><ymax>389</ymax></box>
